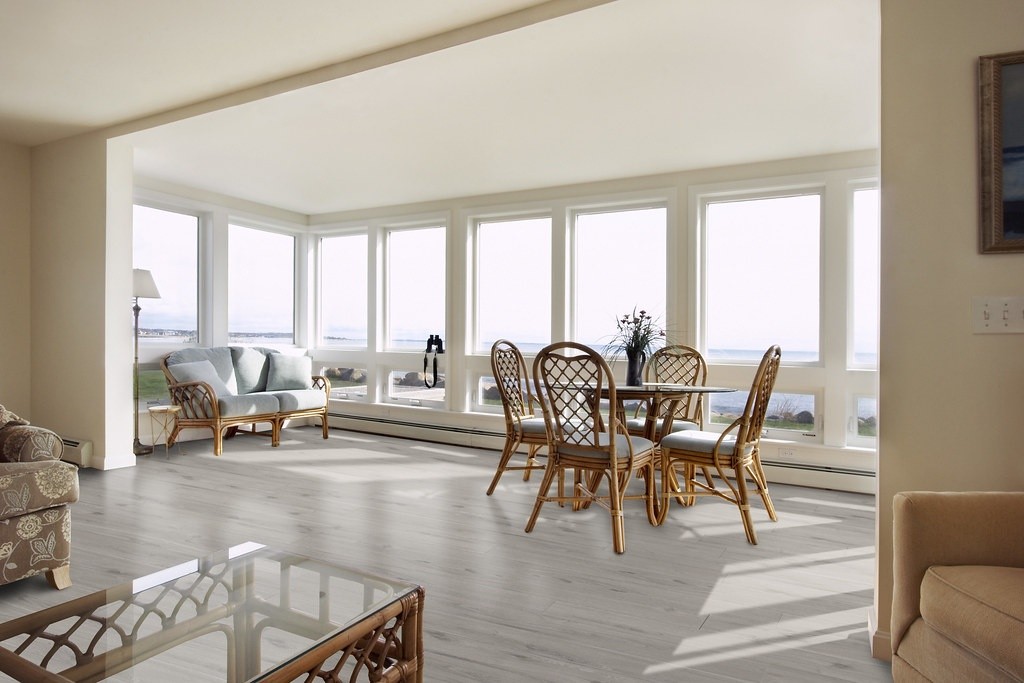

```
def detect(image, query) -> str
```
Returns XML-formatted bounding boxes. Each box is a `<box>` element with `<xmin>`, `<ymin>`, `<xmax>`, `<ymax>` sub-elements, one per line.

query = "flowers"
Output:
<box><xmin>600</xmin><ymin>305</ymin><xmax>686</xmax><ymax>376</ymax></box>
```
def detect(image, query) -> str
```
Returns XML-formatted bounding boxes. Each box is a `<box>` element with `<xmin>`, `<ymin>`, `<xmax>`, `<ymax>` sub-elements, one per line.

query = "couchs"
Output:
<box><xmin>891</xmin><ymin>491</ymin><xmax>1024</xmax><ymax>683</ymax></box>
<box><xmin>0</xmin><ymin>404</ymin><xmax>79</xmax><ymax>590</ymax></box>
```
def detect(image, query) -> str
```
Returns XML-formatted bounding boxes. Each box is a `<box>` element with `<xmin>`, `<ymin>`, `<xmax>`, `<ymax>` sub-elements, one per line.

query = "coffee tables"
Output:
<box><xmin>0</xmin><ymin>541</ymin><xmax>425</xmax><ymax>683</ymax></box>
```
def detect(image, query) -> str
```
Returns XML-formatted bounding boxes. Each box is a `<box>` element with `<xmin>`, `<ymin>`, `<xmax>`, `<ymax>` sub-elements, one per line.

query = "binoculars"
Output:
<box><xmin>426</xmin><ymin>335</ymin><xmax>443</xmax><ymax>354</ymax></box>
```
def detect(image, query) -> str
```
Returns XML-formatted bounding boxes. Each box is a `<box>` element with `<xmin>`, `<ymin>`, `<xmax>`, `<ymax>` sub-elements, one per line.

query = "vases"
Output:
<box><xmin>625</xmin><ymin>349</ymin><xmax>646</xmax><ymax>386</ymax></box>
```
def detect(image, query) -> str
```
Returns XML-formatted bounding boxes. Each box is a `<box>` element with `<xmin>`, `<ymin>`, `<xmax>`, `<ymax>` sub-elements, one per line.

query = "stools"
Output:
<box><xmin>148</xmin><ymin>405</ymin><xmax>182</xmax><ymax>458</ymax></box>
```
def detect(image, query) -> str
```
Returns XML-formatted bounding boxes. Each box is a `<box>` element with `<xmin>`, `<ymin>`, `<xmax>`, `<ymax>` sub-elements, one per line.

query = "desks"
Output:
<box><xmin>540</xmin><ymin>382</ymin><xmax>738</xmax><ymax>509</ymax></box>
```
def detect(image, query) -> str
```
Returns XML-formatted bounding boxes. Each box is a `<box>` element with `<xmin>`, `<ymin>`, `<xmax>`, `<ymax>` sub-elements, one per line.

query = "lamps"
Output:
<box><xmin>132</xmin><ymin>269</ymin><xmax>161</xmax><ymax>455</ymax></box>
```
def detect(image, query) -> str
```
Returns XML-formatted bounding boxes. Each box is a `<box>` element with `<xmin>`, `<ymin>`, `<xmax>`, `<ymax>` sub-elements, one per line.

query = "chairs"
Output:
<box><xmin>486</xmin><ymin>338</ymin><xmax>781</xmax><ymax>554</ymax></box>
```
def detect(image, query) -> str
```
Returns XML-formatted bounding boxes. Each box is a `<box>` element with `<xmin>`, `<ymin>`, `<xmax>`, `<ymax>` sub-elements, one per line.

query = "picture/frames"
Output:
<box><xmin>977</xmin><ymin>50</ymin><xmax>1024</xmax><ymax>254</ymax></box>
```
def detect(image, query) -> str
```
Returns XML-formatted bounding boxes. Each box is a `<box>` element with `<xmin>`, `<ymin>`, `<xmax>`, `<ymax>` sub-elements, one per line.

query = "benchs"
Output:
<box><xmin>160</xmin><ymin>347</ymin><xmax>332</xmax><ymax>456</ymax></box>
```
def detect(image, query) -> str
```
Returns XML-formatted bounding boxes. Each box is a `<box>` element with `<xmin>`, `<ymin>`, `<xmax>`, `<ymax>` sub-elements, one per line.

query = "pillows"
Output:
<box><xmin>266</xmin><ymin>352</ymin><xmax>313</xmax><ymax>390</ymax></box>
<box><xmin>167</xmin><ymin>361</ymin><xmax>231</xmax><ymax>400</ymax></box>
